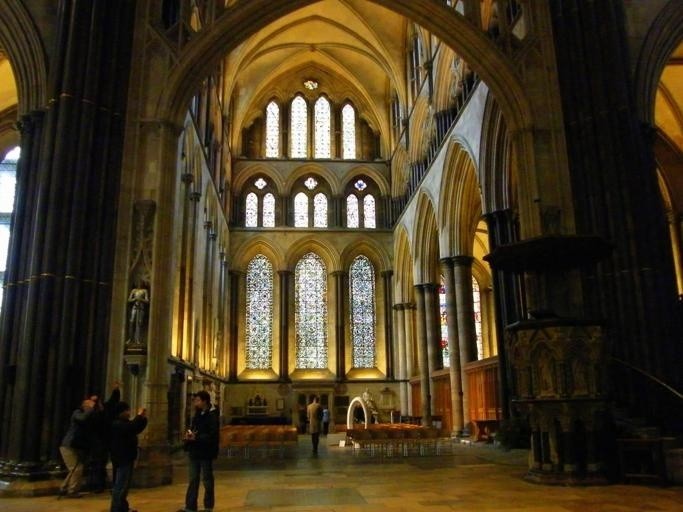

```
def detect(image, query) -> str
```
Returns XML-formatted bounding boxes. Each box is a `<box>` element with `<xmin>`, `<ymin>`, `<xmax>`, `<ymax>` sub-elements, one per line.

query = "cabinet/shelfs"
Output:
<box><xmin>246</xmin><ymin>406</ymin><xmax>268</xmax><ymax>415</ymax></box>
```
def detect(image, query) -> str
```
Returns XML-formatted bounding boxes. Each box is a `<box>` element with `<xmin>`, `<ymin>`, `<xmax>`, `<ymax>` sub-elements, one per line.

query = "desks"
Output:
<box><xmin>472</xmin><ymin>420</ymin><xmax>498</xmax><ymax>444</ymax></box>
<box><xmin>431</xmin><ymin>416</ymin><xmax>443</xmax><ymax>429</ymax></box>
<box><xmin>409</xmin><ymin>417</ymin><xmax>422</xmax><ymax>425</ymax></box>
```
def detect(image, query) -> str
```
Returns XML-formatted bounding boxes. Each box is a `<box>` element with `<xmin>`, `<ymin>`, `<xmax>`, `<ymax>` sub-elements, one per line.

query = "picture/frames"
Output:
<box><xmin>276</xmin><ymin>399</ymin><xmax>285</xmax><ymax>411</ymax></box>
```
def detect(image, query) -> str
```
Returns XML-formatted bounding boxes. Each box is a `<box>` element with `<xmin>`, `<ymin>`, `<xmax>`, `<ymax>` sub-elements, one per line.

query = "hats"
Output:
<box><xmin>116</xmin><ymin>401</ymin><xmax>129</xmax><ymax>416</ymax></box>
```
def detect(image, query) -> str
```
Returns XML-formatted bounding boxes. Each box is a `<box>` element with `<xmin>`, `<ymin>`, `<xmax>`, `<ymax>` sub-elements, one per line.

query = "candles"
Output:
<box><xmin>219</xmin><ymin>245</ymin><xmax>226</xmax><ymax>261</ymax></box>
<box><xmin>211</xmin><ymin>216</ymin><xmax>214</xmax><ymax>234</ymax></box>
<box><xmin>207</xmin><ymin>198</ymin><xmax>210</xmax><ymax>221</ymax></box>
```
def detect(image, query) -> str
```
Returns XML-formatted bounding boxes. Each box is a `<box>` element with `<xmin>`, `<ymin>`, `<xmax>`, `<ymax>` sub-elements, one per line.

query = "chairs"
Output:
<box><xmin>346</xmin><ymin>423</ymin><xmax>452</xmax><ymax>464</ymax></box>
<box><xmin>220</xmin><ymin>425</ymin><xmax>298</xmax><ymax>457</ymax></box>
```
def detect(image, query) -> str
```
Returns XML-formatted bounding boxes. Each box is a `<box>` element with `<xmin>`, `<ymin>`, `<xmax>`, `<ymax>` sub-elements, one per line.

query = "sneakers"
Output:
<box><xmin>127</xmin><ymin>506</ymin><xmax>214</xmax><ymax>512</ymax></box>
<box><xmin>59</xmin><ymin>488</ymin><xmax>82</xmax><ymax>498</ymax></box>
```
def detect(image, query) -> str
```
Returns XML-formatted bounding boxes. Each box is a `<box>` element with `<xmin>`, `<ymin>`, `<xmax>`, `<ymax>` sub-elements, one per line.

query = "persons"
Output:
<box><xmin>57</xmin><ymin>395</ymin><xmax>100</xmax><ymax>499</ymax></box>
<box><xmin>102</xmin><ymin>399</ymin><xmax>148</xmax><ymax>511</ymax></box>
<box><xmin>77</xmin><ymin>379</ymin><xmax>121</xmax><ymax>494</ymax></box>
<box><xmin>175</xmin><ymin>389</ymin><xmax>221</xmax><ymax>512</ymax></box>
<box><xmin>123</xmin><ymin>279</ymin><xmax>149</xmax><ymax>345</ymax></box>
<box><xmin>321</xmin><ymin>404</ymin><xmax>330</xmax><ymax>435</ymax></box>
<box><xmin>305</xmin><ymin>396</ymin><xmax>323</xmax><ymax>458</ymax></box>
